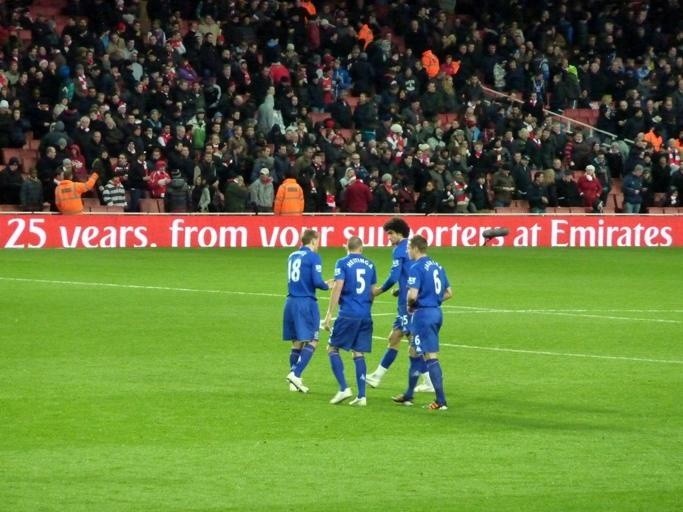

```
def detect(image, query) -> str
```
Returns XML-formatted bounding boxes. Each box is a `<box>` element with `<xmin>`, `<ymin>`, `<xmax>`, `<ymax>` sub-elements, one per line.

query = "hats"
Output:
<box><xmin>0</xmin><ymin>100</ymin><xmax>8</xmax><ymax>108</ymax></box>
<box><xmin>384</xmin><ymin>114</ymin><xmax>402</xmax><ymax>132</ymax></box>
<box><xmin>259</xmin><ymin>168</ymin><xmax>269</xmax><ymax>174</ymax></box>
<box><xmin>314</xmin><ymin>120</ymin><xmax>335</xmax><ymax>129</ymax></box>
<box><xmin>381</xmin><ymin>173</ymin><xmax>392</xmax><ymax>182</ymax></box>
<box><xmin>155</xmin><ymin>161</ymin><xmax>166</xmax><ymax>169</ymax></box>
<box><xmin>419</xmin><ymin>143</ymin><xmax>430</xmax><ymax>151</ymax></box>
<box><xmin>453</xmin><ymin>129</ymin><xmax>464</xmax><ymax>137</ymax></box>
<box><xmin>522</xmin><ymin>155</ymin><xmax>530</xmax><ymax>160</ymax></box>
<box><xmin>171</xmin><ymin>168</ymin><xmax>182</xmax><ymax>178</ymax></box>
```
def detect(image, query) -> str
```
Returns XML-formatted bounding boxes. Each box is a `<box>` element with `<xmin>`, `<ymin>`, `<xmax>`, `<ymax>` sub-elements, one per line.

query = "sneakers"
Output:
<box><xmin>415</xmin><ymin>383</ymin><xmax>435</xmax><ymax>392</ymax></box>
<box><xmin>349</xmin><ymin>397</ymin><xmax>366</xmax><ymax>405</ymax></box>
<box><xmin>392</xmin><ymin>394</ymin><xmax>412</xmax><ymax>405</ymax></box>
<box><xmin>287</xmin><ymin>371</ymin><xmax>308</xmax><ymax>392</ymax></box>
<box><xmin>366</xmin><ymin>374</ymin><xmax>380</xmax><ymax>388</ymax></box>
<box><xmin>330</xmin><ymin>388</ymin><xmax>352</xmax><ymax>403</ymax></box>
<box><xmin>422</xmin><ymin>401</ymin><xmax>447</xmax><ymax>410</ymax></box>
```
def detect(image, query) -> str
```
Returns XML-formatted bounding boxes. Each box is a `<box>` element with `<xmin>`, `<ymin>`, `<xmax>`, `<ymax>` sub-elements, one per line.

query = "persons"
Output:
<box><xmin>1</xmin><ymin>1</ymin><xmax>682</xmax><ymax>212</ymax></box>
<box><xmin>321</xmin><ymin>236</ymin><xmax>377</xmax><ymax>406</ymax></box>
<box><xmin>391</xmin><ymin>234</ymin><xmax>453</xmax><ymax>409</ymax></box>
<box><xmin>283</xmin><ymin>230</ymin><xmax>335</xmax><ymax>392</ymax></box>
<box><xmin>365</xmin><ymin>218</ymin><xmax>435</xmax><ymax>392</ymax></box>
